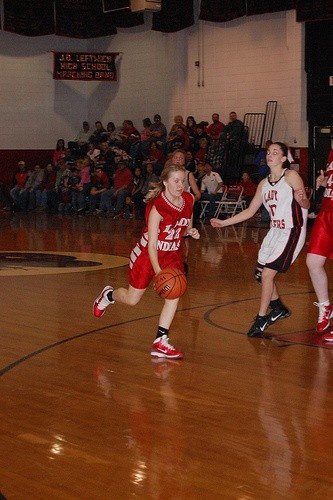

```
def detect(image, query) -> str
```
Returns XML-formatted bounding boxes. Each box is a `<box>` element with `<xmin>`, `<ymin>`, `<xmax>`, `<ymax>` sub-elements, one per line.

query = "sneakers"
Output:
<box><xmin>150</xmin><ymin>335</ymin><xmax>183</xmax><ymax>358</ymax></box>
<box><xmin>247</xmin><ymin>314</ymin><xmax>270</xmax><ymax>337</ymax></box>
<box><xmin>92</xmin><ymin>286</ymin><xmax>115</xmax><ymax>319</ymax></box>
<box><xmin>267</xmin><ymin>304</ymin><xmax>290</xmax><ymax>325</ymax></box>
<box><xmin>313</xmin><ymin>302</ymin><xmax>333</xmax><ymax>333</ymax></box>
<box><xmin>321</xmin><ymin>331</ymin><xmax>333</xmax><ymax>342</ymax></box>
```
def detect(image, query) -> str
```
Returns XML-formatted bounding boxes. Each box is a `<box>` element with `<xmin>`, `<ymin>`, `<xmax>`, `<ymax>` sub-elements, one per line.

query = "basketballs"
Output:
<box><xmin>153</xmin><ymin>268</ymin><xmax>188</xmax><ymax>299</ymax></box>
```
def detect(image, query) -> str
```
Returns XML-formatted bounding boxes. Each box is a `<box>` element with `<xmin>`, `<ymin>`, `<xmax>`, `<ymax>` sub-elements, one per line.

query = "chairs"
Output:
<box><xmin>199</xmin><ymin>184</ymin><xmax>247</xmax><ymax>220</ymax></box>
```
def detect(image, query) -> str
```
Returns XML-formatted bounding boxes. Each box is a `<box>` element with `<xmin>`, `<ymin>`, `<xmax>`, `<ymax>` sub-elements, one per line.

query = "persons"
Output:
<box><xmin>145</xmin><ymin>149</ymin><xmax>201</xmax><ymax>277</ymax></box>
<box><xmin>209</xmin><ymin>142</ymin><xmax>311</xmax><ymax>337</ymax></box>
<box><xmin>92</xmin><ymin>164</ymin><xmax>196</xmax><ymax>359</ymax></box>
<box><xmin>305</xmin><ymin>149</ymin><xmax>333</xmax><ymax>342</ymax></box>
<box><xmin>4</xmin><ymin>112</ymin><xmax>299</xmax><ymax>232</ymax></box>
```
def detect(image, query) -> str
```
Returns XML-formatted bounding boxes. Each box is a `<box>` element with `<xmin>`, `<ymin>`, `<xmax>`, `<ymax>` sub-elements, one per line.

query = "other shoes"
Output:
<box><xmin>183</xmin><ymin>262</ymin><xmax>188</xmax><ymax>273</ymax></box>
